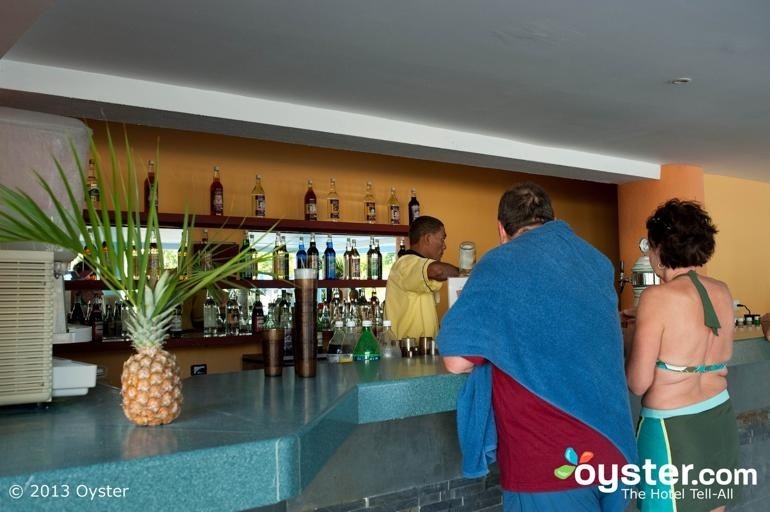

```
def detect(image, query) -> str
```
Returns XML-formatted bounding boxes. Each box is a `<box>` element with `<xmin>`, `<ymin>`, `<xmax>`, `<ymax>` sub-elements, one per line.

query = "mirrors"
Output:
<box><xmin>63</xmin><ymin>287</ymin><xmax>394</xmax><ymax>338</ymax></box>
<box><xmin>66</xmin><ymin>225</ymin><xmax>411</xmax><ymax>283</ymax></box>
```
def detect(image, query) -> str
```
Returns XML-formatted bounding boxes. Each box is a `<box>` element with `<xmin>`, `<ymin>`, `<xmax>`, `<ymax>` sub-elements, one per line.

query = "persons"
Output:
<box><xmin>383</xmin><ymin>215</ymin><xmax>473</xmax><ymax>344</ymax></box>
<box><xmin>628</xmin><ymin>196</ymin><xmax>739</xmax><ymax>512</ymax></box>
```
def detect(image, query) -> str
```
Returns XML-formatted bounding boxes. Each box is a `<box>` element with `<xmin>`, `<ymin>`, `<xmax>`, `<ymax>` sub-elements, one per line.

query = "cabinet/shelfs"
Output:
<box><xmin>65</xmin><ymin>209</ymin><xmax>417</xmax><ymax>356</ymax></box>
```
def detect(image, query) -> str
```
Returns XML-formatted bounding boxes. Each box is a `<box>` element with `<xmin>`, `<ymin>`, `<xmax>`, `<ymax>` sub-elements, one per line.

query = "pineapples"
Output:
<box><xmin>120</xmin><ymin>267</ymin><xmax>184</xmax><ymax>427</ymax></box>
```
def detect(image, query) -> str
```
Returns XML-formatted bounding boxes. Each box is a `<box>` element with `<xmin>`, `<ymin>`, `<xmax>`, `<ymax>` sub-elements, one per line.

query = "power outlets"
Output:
<box><xmin>732</xmin><ymin>300</ymin><xmax>746</xmax><ymax>309</ymax></box>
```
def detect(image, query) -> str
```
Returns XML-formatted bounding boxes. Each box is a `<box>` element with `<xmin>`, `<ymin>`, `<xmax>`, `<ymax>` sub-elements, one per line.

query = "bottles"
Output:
<box><xmin>143</xmin><ymin>160</ymin><xmax>159</xmax><ymax>213</ymax></box>
<box><xmin>208</xmin><ymin>167</ymin><xmax>224</xmax><ymax>216</ymax></box>
<box><xmin>69</xmin><ymin>230</ymin><xmax>405</xmax><ymax>340</ymax></box>
<box><xmin>84</xmin><ymin>159</ymin><xmax>102</xmax><ymax>209</ymax></box>
<box><xmin>459</xmin><ymin>241</ymin><xmax>477</xmax><ymax>277</ymax></box>
<box><xmin>303</xmin><ymin>179</ymin><xmax>318</xmax><ymax>221</ymax></box>
<box><xmin>401</xmin><ymin>336</ymin><xmax>433</xmax><ymax>357</ymax></box>
<box><xmin>734</xmin><ymin>316</ymin><xmax>760</xmax><ymax>329</ymax></box>
<box><xmin>326</xmin><ymin>178</ymin><xmax>341</xmax><ymax>222</ymax></box>
<box><xmin>326</xmin><ymin>319</ymin><xmax>403</xmax><ymax>365</ymax></box>
<box><xmin>408</xmin><ymin>188</ymin><xmax>421</xmax><ymax>226</ymax></box>
<box><xmin>385</xmin><ymin>186</ymin><xmax>401</xmax><ymax>225</ymax></box>
<box><xmin>362</xmin><ymin>180</ymin><xmax>377</xmax><ymax>224</ymax></box>
<box><xmin>250</xmin><ymin>174</ymin><xmax>265</xmax><ymax>218</ymax></box>
